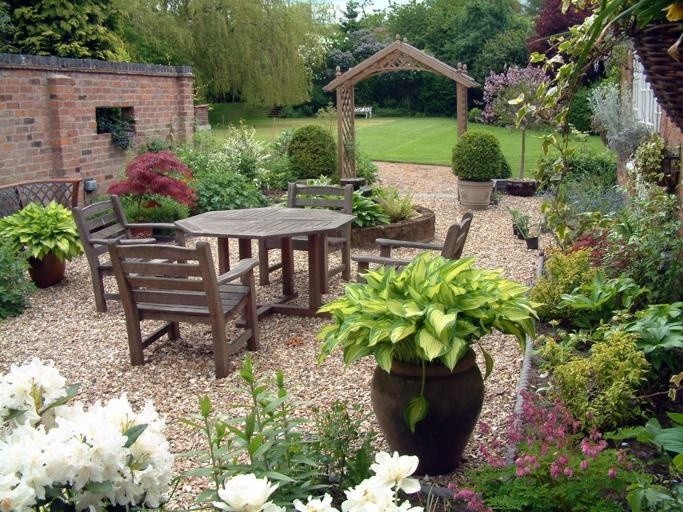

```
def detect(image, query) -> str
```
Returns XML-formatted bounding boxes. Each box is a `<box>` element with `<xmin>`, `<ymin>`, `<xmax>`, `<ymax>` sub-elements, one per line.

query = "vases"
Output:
<box><xmin>629</xmin><ymin>20</ymin><xmax>683</xmax><ymax>133</ymax></box>
<box><xmin>506</xmin><ymin>179</ymin><xmax>535</xmax><ymax>196</ymax></box>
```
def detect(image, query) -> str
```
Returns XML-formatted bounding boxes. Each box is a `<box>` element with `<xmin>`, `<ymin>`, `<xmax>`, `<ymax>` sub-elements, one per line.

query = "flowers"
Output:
<box><xmin>473</xmin><ymin>62</ymin><xmax>570</xmax><ymax>178</ymax></box>
<box><xmin>524</xmin><ymin>0</ymin><xmax>683</xmax><ymax>236</ymax></box>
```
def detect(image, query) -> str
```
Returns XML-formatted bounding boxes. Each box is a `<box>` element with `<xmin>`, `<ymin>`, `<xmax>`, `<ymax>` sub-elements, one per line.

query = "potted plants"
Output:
<box><xmin>505</xmin><ymin>206</ymin><xmax>541</xmax><ymax>249</ymax></box>
<box><xmin>316</xmin><ymin>248</ymin><xmax>551</xmax><ymax>476</ymax></box>
<box><xmin>106</xmin><ymin>150</ymin><xmax>199</xmax><ymax>238</ymax></box>
<box><xmin>452</xmin><ymin>129</ymin><xmax>502</xmax><ymax>211</ymax></box>
<box><xmin>139</xmin><ymin>201</ymin><xmax>189</xmax><ymax>242</ymax></box>
<box><xmin>0</xmin><ymin>199</ymin><xmax>85</xmax><ymax>289</ymax></box>
<box><xmin>632</xmin><ymin>133</ymin><xmax>680</xmax><ymax>194</ymax></box>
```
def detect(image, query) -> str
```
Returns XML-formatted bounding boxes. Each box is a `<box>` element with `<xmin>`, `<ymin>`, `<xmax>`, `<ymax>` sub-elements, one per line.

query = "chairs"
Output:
<box><xmin>351</xmin><ymin>212</ymin><xmax>473</xmax><ymax>284</ymax></box>
<box><xmin>72</xmin><ymin>194</ymin><xmax>188</xmax><ymax>312</ymax></box>
<box><xmin>259</xmin><ymin>183</ymin><xmax>354</xmax><ymax>294</ymax></box>
<box><xmin>108</xmin><ymin>239</ymin><xmax>260</xmax><ymax>378</ymax></box>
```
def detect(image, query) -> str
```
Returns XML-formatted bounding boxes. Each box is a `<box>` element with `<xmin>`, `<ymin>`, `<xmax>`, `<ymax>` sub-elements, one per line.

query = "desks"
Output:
<box><xmin>174</xmin><ymin>207</ymin><xmax>358</xmax><ymax>328</ymax></box>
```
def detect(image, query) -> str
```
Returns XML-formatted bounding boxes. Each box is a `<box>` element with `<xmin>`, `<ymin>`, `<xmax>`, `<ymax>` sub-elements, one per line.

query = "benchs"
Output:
<box><xmin>354</xmin><ymin>106</ymin><xmax>372</xmax><ymax>119</ymax></box>
